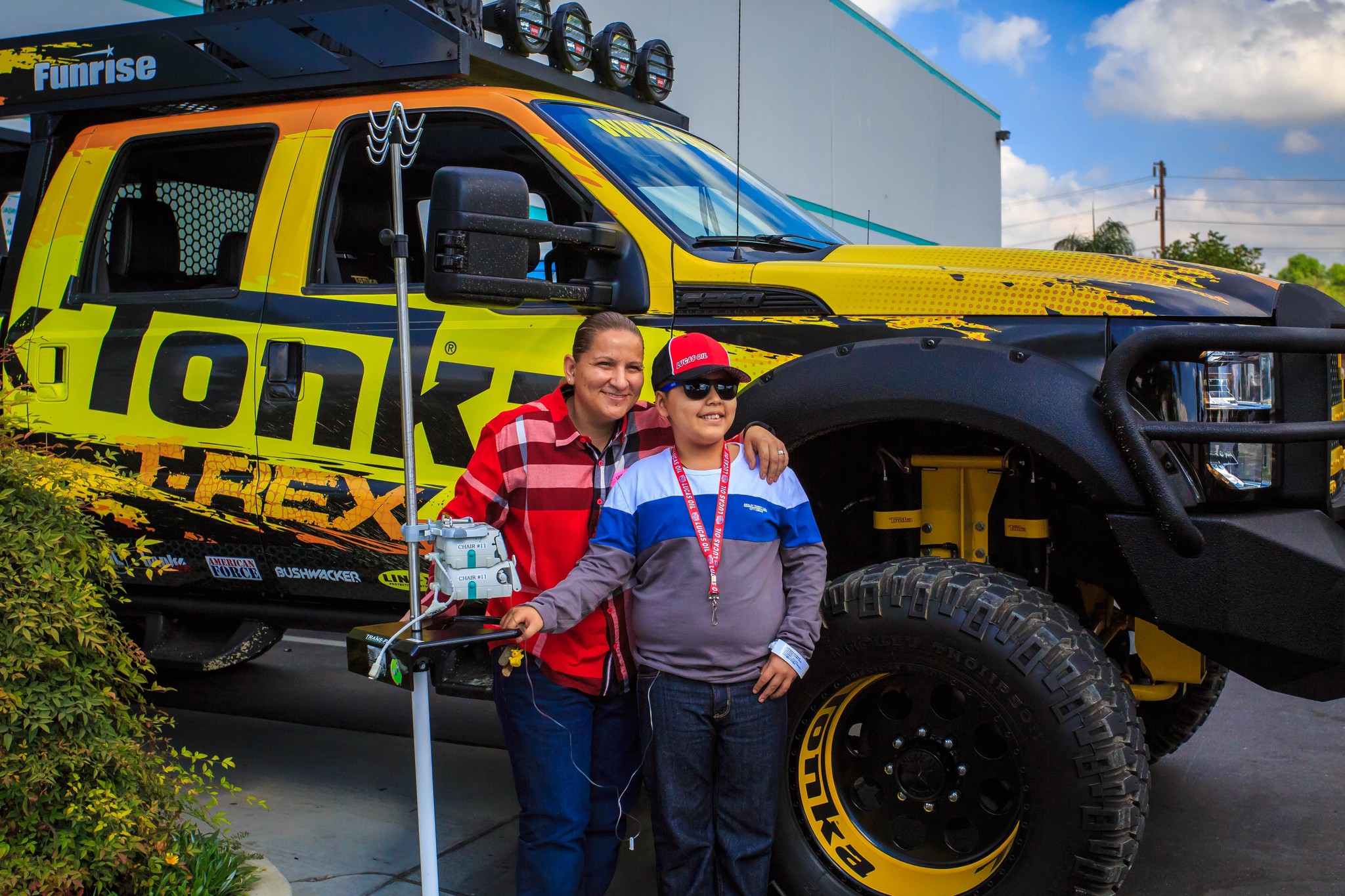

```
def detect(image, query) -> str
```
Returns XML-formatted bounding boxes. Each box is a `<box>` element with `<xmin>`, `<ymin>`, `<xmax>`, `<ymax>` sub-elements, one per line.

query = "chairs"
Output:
<box><xmin>109</xmin><ymin>197</ymin><xmax>182</xmax><ymax>293</ymax></box>
<box><xmin>322</xmin><ymin>192</ymin><xmax>397</xmax><ymax>286</ymax></box>
<box><xmin>212</xmin><ymin>231</ymin><xmax>247</xmax><ymax>288</ymax></box>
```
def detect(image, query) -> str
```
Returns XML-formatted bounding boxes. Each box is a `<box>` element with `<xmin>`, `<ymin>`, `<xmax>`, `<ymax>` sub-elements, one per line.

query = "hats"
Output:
<box><xmin>651</xmin><ymin>332</ymin><xmax>752</xmax><ymax>392</ymax></box>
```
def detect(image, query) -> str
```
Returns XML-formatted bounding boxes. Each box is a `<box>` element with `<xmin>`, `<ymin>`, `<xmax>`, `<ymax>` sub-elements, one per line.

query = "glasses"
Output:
<box><xmin>660</xmin><ymin>378</ymin><xmax>739</xmax><ymax>400</ymax></box>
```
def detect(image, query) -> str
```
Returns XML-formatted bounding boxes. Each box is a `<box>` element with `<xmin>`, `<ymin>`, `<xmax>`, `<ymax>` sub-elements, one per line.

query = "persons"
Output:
<box><xmin>500</xmin><ymin>333</ymin><xmax>828</xmax><ymax>896</ymax></box>
<box><xmin>379</xmin><ymin>313</ymin><xmax>790</xmax><ymax>896</ymax></box>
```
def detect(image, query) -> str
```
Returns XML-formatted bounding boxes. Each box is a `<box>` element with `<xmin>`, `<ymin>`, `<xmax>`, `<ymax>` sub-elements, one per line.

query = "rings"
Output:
<box><xmin>778</xmin><ymin>450</ymin><xmax>786</xmax><ymax>455</ymax></box>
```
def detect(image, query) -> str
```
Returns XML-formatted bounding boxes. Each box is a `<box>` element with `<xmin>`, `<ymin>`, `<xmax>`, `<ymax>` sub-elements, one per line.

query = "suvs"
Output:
<box><xmin>0</xmin><ymin>0</ymin><xmax>1345</xmax><ymax>896</ymax></box>
